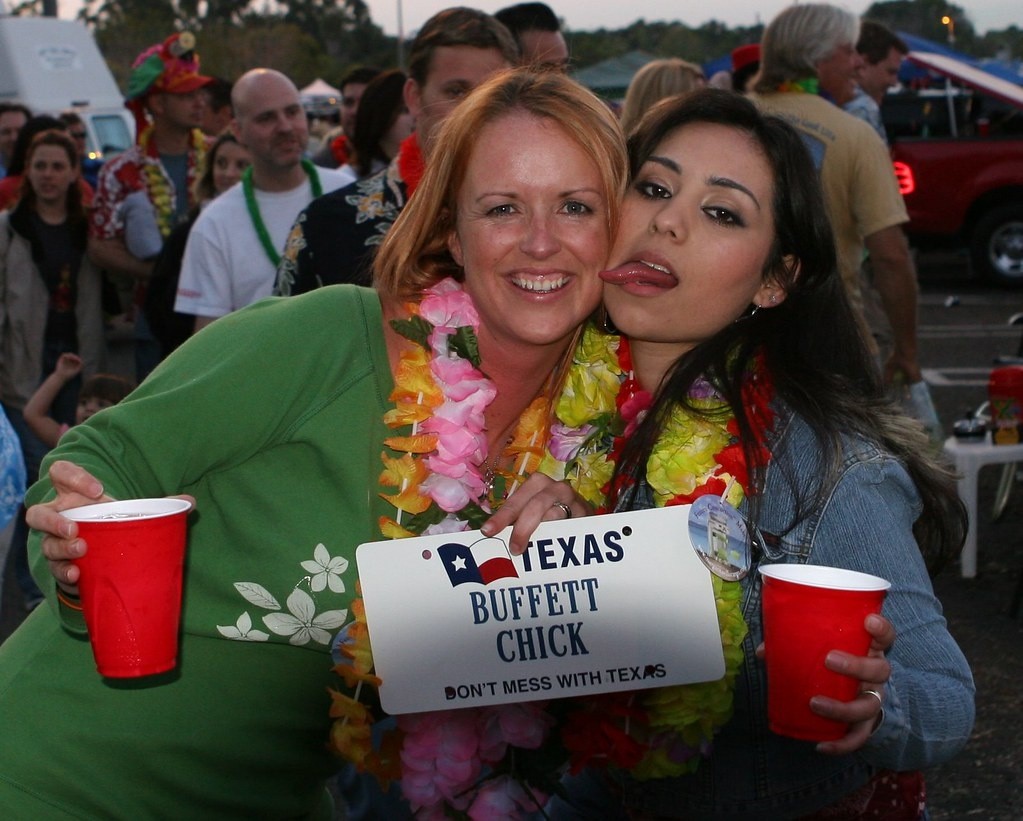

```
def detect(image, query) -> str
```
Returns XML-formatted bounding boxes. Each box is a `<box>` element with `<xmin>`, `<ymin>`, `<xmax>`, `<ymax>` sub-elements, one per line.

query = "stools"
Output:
<box><xmin>936</xmin><ymin>432</ymin><xmax>1023</xmax><ymax>577</ymax></box>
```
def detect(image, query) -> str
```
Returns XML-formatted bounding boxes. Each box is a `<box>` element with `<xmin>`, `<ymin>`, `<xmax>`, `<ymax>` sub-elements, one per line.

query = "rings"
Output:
<box><xmin>863</xmin><ymin>689</ymin><xmax>882</xmax><ymax>701</ymax></box>
<box><xmin>553</xmin><ymin>501</ymin><xmax>573</xmax><ymax>519</ymax></box>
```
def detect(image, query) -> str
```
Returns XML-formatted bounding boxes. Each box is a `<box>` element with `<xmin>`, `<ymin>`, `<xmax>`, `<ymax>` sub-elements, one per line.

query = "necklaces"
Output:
<box><xmin>483</xmin><ymin>440</ymin><xmax>511</xmax><ymax>485</ymax></box>
<box><xmin>134</xmin><ymin>126</ymin><xmax>206</xmax><ymax>237</ymax></box>
<box><xmin>330</xmin><ymin>296</ymin><xmax>552</xmax><ymax>780</ymax></box>
<box><xmin>397</xmin><ymin>328</ymin><xmax>776</xmax><ymax>821</ymax></box>
<box><xmin>242</xmin><ymin>156</ymin><xmax>322</xmax><ymax>268</ymax></box>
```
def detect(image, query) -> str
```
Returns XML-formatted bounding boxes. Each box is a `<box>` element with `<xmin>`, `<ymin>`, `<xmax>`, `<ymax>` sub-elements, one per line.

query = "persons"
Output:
<box><xmin>0</xmin><ymin>0</ymin><xmax>976</xmax><ymax>820</ymax></box>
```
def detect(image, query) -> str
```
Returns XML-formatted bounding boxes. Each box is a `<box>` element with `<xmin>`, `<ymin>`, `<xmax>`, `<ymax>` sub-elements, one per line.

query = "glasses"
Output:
<box><xmin>70</xmin><ymin>130</ymin><xmax>86</xmax><ymax>138</ymax></box>
<box><xmin>342</xmin><ymin>97</ymin><xmax>360</xmax><ymax>110</ymax></box>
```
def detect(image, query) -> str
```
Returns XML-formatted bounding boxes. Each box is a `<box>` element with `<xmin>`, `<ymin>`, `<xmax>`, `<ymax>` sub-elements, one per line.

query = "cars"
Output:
<box><xmin>876</xmin><ymin>50</ymin><xmax>1023</xmax><ymax>287</ymax></box>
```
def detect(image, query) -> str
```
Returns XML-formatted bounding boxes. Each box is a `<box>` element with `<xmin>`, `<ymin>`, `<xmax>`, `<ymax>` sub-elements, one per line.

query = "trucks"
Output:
<box><xmin>1</xmin><ymin>16</ymin><xmax>140</xmax><ymax>161</ymax></box>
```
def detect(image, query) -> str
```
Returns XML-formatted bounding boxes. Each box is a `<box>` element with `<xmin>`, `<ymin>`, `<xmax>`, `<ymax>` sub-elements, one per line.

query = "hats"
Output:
<box><xmin>124</xmin><ymin>32</ymin><xmax>212</xmax><ymax>98</ymax></box>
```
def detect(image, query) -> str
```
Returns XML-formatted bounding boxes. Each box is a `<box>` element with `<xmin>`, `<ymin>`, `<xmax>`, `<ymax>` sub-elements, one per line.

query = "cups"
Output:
<box><xmin>56</xmin><ymin>498</ymin><xmax>193</xmax><ymax>679</ymax></box>
<box><xmin>758</xmin><ymin>563</ymin><xmax>892</xmax><ymax>745</ymax></box>
